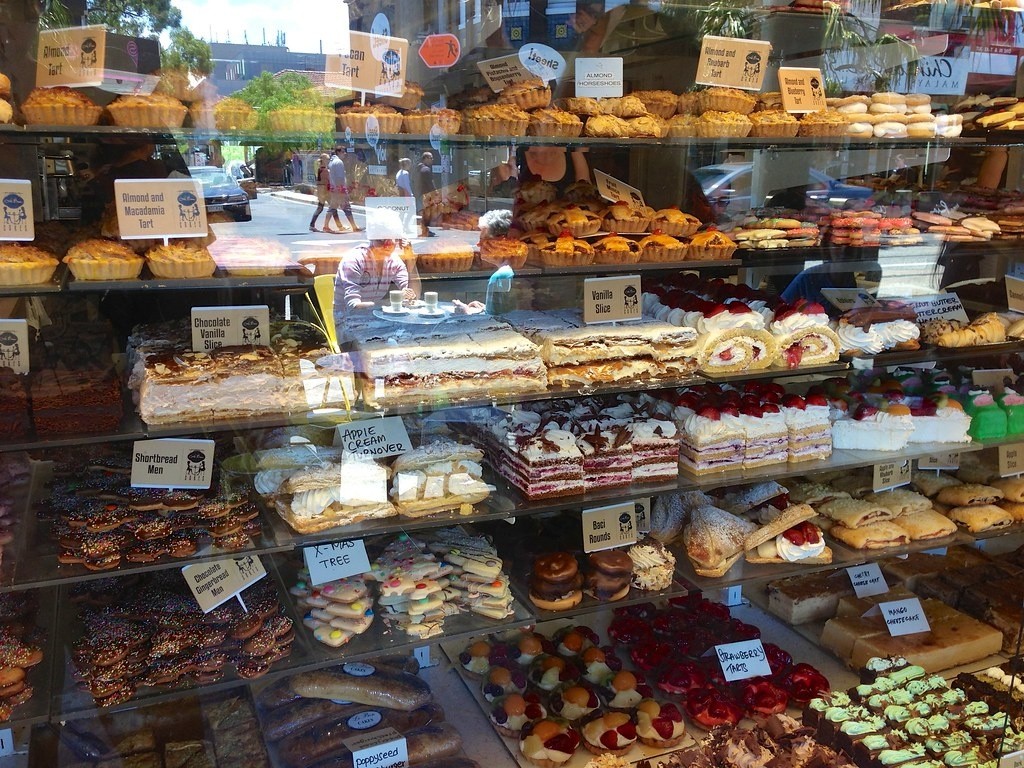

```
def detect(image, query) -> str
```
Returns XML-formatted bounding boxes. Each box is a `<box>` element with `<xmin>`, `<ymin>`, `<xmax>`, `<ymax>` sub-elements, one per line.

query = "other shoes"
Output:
<box><xmin>309</xmin><ymin>226</ymin><xmax>320</xmax><ymax>231</ymax></box>
<box><xmin>339</xmin><ymin>227</ymin><xmax>351</xmax><ymax>231</ymax></box>
<box><xmin>422</xmin><ymin>230</ymin><xmax>435</xmax><ymax>236</ymax></box>
<box><xmin>353</xmin><ymin>227</ymin><xmax>366</xmax><ymax>232</ymax></box>
<box><xmin>323</xmin><ymin>228</ymin><xmax>335</xmax><ymax>233</ymax></box>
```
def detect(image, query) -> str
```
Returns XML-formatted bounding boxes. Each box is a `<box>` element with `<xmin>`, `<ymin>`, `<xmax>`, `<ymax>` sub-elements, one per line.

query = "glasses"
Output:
<box><xmin>428</xmin><ymin>157</ymin><xmax>433</xmax><ymax>159</ymax></box>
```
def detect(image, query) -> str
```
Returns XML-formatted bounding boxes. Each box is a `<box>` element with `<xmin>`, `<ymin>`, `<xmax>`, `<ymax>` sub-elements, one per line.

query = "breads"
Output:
<box><xmin>0</xmin><ymin>72</ymin><xmax>1024</xmax><ymax>283</ymax></box>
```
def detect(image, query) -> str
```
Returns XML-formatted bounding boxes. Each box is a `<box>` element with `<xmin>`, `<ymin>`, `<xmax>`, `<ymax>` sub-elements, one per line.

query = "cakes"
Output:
<box><xmin>0</xmin><ymin>270</ymin><xmax>1024</xmax><ymax>767</ymax></box>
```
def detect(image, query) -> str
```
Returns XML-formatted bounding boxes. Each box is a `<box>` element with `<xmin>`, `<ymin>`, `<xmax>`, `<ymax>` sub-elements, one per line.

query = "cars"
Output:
<box><xmin>186</xmin><ymin>166</ymin><xmax>252</xmax><ymax>222</ymax></box>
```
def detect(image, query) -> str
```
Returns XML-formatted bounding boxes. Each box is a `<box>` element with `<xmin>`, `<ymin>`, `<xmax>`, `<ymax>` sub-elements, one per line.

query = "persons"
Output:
<box><xmin>108</xmin><ymin>0</ymin><xmax>1014</xmax><ymax>670</ymax></box>
<box><xmin>79</xmin><ymin>141</ymin><xmax>179</xmax><ymax>228</ymax></box>
<box><xmin>487</xmin><ymin>143</ymin><xmax>592</xmax><ymax>190</ymax></box>
<box><xmin>932</xmin><ymin>147</ymin><xmax>1009</xmax><ymax>308</ymax></box>
